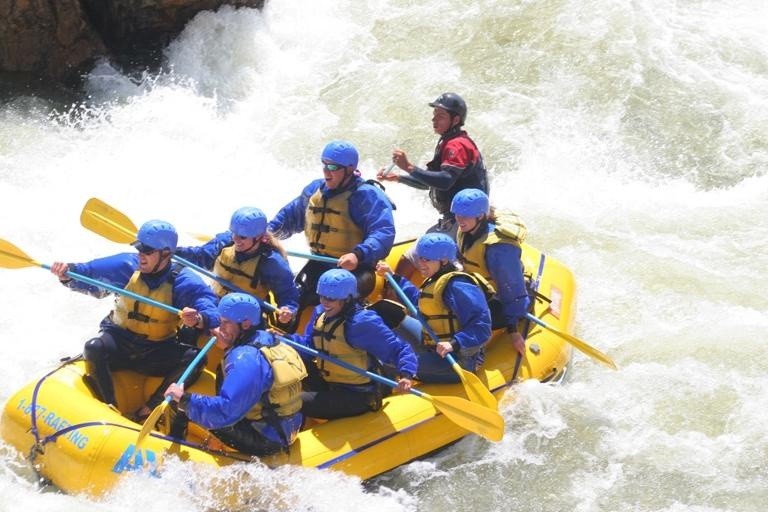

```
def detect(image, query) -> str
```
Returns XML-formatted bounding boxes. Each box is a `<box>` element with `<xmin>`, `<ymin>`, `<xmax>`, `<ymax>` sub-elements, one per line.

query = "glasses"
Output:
<box><xmin>322</xmin><ymin>161</ymin><xmax>341</xmax><ymax>171</ymax></box>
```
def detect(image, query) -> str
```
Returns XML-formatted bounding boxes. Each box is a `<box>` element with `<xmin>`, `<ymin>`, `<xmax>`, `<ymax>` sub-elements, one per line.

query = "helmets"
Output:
<box><xmin>428</xmin><ymin>92</ymin><xmax>467</xmax><ymax>117</ymax></box>
<box><xmin>448</xmin><ymin>188</ymin><xmax>489</xmax><ymax>218</ymax></box>
<box><xmin>322</xmin><ymin>141</ymin><xmax>358</xmax><ymax>170</ymax></box>
<box><xmin>315</xmin><ymin>269</ymin><xmax>358</xmax><ymax>298</ymax></box>
<box><xmin>227</xmin><ymin>208</ymin><xmax>267</xmax><ymax>238</ymax></box>
<box><xmin>137</xmin><ymin>219</ymin><xmax>178</xmax><ymax>253</ymax></box>
<box><xmin>218</xmin><ymin>291</ymin><xmax>262</xmax><ymax>328</ymax></box>
<box><xmin>415</xmin><ymin>235</ymin><xmax>456</xmax><ymax>262</ymax></box>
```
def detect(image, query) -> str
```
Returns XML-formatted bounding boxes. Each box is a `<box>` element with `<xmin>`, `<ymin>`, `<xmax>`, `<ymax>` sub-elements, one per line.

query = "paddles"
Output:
<box><xmin>0</xmin><ymin>239</ymin><xmax>182</xmax><ymax>319</ymax></box>
<box><xmin>384</xmin><ymin>271</ymin><xmax>497</xmax><ymax>413</ymax></box>
<box><xmin>274</xmin><ymin>334</ymin><xmax>505</xmax><ymax>442</ymax></box>
<box><xmin>493</xmin><ymin>295</ymin><xmax>620</xmax><ymax>371</ymax></box>
<box><xmin>135</xmin><ymin>336</ymin><xmax>218</xmax><ymax>453</ymax></box>
<box><xmin>81</xmin><ymin>198</ymin><xmax>282</xmax><ymax>318</ymax></box>
<box><xmin>197</xmin><ymin>234</ymin><xmax>342</xmax><ymax>264</ymax></box>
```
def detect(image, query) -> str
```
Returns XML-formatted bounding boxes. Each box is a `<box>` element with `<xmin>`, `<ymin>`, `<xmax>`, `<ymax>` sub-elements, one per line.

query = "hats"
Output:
<box><xmin>131</xmin><ymin>239</ymin><xmax>154</xmax><ymax>252</ymax></box>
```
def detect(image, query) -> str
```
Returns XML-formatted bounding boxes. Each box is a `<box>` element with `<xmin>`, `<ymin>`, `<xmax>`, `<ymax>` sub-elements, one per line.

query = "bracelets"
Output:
<box><xmin>193</xmin><ymin>314</ymin><xmax>201</xmax><ymax>327</ymax></box>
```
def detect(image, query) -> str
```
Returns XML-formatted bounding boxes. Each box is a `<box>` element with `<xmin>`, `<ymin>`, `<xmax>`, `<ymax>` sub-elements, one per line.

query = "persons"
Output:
<box><xmin>175</xmin><ymin>207</ymin><xmax>300</xmax><ymax>348</ymax></box>
<box><xmin>267</xmin><ymin>141</ymin><xmax>396</xmax><ymax>331</ymax></box>
<box><xmin>376</xmin><ymin>233</ymin><xmax>493</xmax><ymax>399</ymax></box>
<box><xmin>450</xmin><ymin>189</ymin><xmax>531</xmax><ymax>356</ymax></box>
<box><xmin>377</xmin><ymin>92</ymin><xmax>490</xmax><ymax>302</ymax></box>
<box><xmin>164</xmin><ymin>293</ymin><xmax>309</xmax><ymax>457</ymax></box>
<box><xmin>266</xmin><ymin>269</ymin><xmax>418</xmax><ymax>432</ymax></box>
<box><xmin>50</xmin><ymin>219</ymin><xmax>220</xmax><ymax>432</ymax></box>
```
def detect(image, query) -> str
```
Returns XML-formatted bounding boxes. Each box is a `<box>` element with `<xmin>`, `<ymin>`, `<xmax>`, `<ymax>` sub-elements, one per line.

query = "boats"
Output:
<box><xmin>2</xmin><ymin>230</ymin><xmax>577</xmax><ymax>512</ymax></box>
